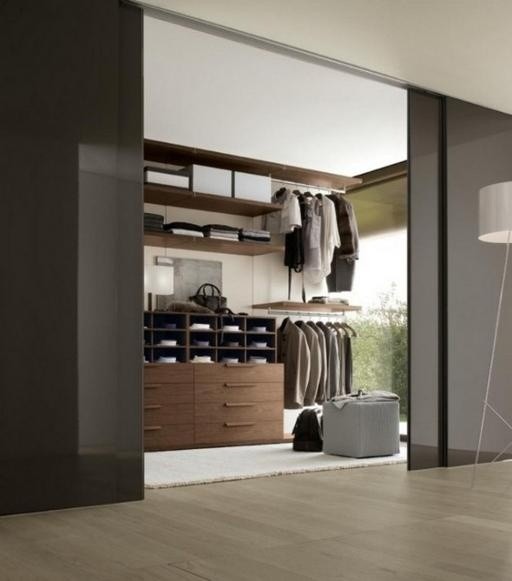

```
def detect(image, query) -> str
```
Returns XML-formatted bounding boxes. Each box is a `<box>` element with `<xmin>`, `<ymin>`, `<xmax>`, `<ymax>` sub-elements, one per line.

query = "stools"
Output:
<box><xmin>323</xmin><ymin>401</ymin><xmax>400</xmax><ymax>457</ymax></box>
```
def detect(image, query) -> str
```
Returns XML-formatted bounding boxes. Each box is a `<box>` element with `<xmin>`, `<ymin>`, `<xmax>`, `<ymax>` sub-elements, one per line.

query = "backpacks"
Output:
<box><xmin>293</xmin><ymin>409</ymin><xmax>323</xmax><ymax>451</ymax></box>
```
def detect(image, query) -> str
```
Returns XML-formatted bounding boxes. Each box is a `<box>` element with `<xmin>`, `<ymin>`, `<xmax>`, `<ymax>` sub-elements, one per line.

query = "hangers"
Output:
<box><xmin>276</xmin><ymin>180</ymin><xmax>340</xmax><ymax>199</ymax></box>
<box><xmin>279</xmin><ymin>310</ymin><xmax>359</xmax><ymax>338</ymax></box>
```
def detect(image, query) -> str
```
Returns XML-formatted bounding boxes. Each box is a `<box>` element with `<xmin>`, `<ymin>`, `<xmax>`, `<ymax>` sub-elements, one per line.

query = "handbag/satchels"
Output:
<box><xmin>189</xmin><ymin>283</ymin><xmax>227</xmax><ymax>313</ymax></box>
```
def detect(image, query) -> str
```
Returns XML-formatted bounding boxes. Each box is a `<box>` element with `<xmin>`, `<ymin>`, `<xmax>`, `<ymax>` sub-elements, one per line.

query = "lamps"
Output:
<box><xmin>144</xmin><ymin>258</ymin><xmax>174</xmax><ymax>311</ymax></box>
<box><xmin>473</xmin><ymin>181</ymin><xmax>512</xmax><ymax>472</ymax></box>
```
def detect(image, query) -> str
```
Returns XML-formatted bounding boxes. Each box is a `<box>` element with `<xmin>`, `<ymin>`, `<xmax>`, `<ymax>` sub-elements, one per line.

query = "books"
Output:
<box><xmin>308</xmin><ymin>297</ymin><xmax>350</xmax><ymax>306</ymax></box>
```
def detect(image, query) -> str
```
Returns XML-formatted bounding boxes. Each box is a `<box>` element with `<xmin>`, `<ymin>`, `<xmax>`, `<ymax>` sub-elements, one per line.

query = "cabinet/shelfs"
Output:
<box><xmin>143</xmin><ymin>312</ymin><xmax>278</xmax><ymax>364</ymax></box>
<box><xmin>143</xmin><ymin>137</ymin><xmax>362</xmax><ymax>256</ymax></box>
<box><xmin>143</xmin><ymin>363</ymin><xmax>295</xmax><ymax>453</ymax></box>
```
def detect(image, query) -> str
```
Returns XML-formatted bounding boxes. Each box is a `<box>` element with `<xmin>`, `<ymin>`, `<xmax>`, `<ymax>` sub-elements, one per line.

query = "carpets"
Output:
<box><xmin>144</xmin><ymin>442</ymin><xmax>408</xmax><ymax>488</ymax></box>
